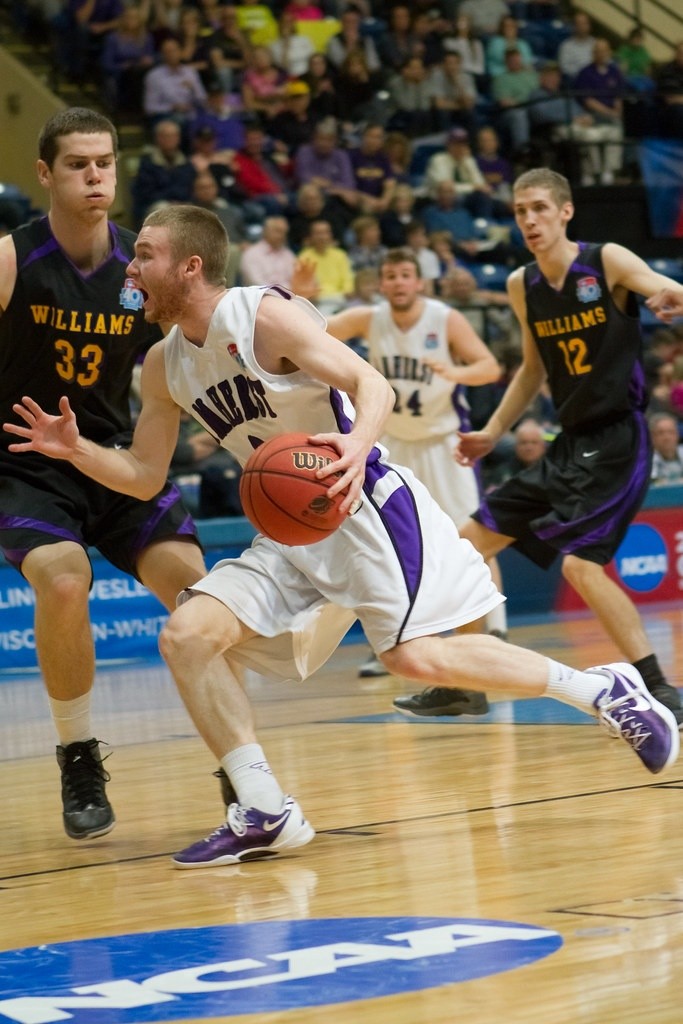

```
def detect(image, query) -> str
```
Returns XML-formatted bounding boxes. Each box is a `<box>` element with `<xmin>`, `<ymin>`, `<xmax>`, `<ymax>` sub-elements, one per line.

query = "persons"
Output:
<box><xmin>3</xmin><ymin>207</ymin><xmax>680</xmax><ymax>868</ymax></box>
<box><xmin>0</xmin><ymin>107</ymin><xmax>254</xmax><ymax>841</ymax></box>
<box><xmin>326</xmin><ymin>252</ymin><xmax>505</xmax><ymax>678</ymax></box>
<box><xmin>0</xmin><ymin>0</ymin><xmax>683</xmax><ymax>495</ymax></box>
<box><xmin>392</xmin><ymin>167</ymin><xmax>683</xmax><ymax>728</ymax></box>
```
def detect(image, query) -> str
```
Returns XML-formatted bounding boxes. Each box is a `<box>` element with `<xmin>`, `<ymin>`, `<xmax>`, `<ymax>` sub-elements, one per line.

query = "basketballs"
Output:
<box><xmin>237</xmin><ymin>431</ymin><xmax>356</xmax><ymax>549</ymax></box>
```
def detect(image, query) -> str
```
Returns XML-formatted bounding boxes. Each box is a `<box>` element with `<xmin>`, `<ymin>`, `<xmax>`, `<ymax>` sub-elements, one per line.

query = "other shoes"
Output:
<box><xmin>359</xmin><ymin>653</ymin><xmax>389</xmax><ymax>676</ymax></box>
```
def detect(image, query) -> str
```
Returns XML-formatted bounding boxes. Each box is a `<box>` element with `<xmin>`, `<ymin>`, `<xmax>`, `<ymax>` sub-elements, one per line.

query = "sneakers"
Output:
<box><xmin>170</xmin><ymin>794</ymin><xmax>317</xmax><ymax>869</ymax></box>
<box><xmin>394</xmin><ymin>685</ymin><xmax>489</xmax><ymax>719</ymax></box>
<box><xmin>55</xmin><ymin>738</ymin><xmax>118</xmax><ymax>841</ymax></box>
<box><xmin>582</xmin><ymin>661</ymin><xmax>680</xmax><ymax>773</ymax></box>
<box><xmin>646</xmin><ymin>683</ymin><xmax>683</xmax><ymax>730</ymax></box>
<box><xmin>213</xmin><ymin>765</ymin><xmax>241</xmax><ymax>808</ymax></box>
